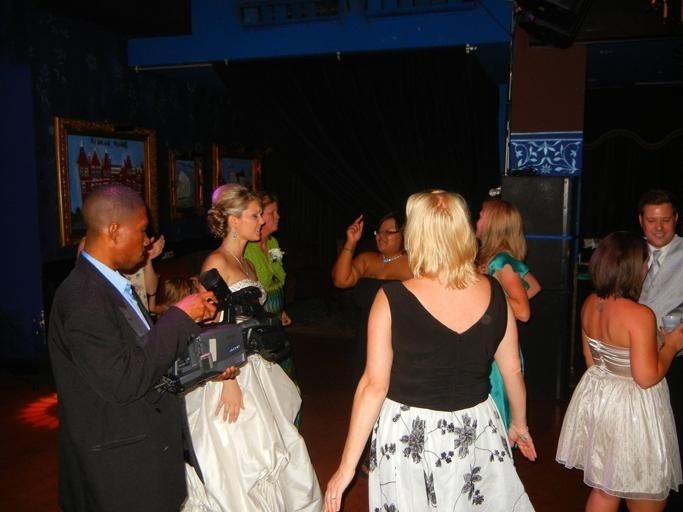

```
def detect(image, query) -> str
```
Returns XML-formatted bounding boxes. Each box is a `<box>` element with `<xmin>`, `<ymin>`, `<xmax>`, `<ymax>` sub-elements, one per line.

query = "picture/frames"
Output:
<box><xmin>168</xmin><ymin>147</ymin><xmax>206</xmax><ymax>223</ymax></box>
<box><xmin>211</xmin><ymin>143</ymin><xmax>262</xmax><ymax>193</ymax></box>
<box><xmin>55</xmin><ymin>116</ymin><xmax>160</xmax><ymax>249</ymax></box>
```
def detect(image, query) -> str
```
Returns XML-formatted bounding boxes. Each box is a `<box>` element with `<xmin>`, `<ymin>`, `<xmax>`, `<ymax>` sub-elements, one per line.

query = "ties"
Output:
<box><xmin>636</xmin><ymin>251</ymin><xmax>661</xmax><ymax>309</ymax></box>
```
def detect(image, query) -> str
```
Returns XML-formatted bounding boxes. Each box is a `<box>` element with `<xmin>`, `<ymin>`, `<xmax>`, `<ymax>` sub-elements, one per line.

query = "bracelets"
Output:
<box><xmin>342</xmin><ymin>247</ymin><xmax>355</xmax><ymax>252</ymax></box>
<box><xmin>509</xmin><ymin>424</ymin><xmax>529</xmax><ymax>435</ymax></box>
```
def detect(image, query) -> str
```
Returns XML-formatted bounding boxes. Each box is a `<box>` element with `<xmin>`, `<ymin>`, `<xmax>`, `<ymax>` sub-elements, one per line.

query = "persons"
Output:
<box><xmin>332</xmin><ymin>210</ymin><xmax>414</xmax><ymax>479</ymax></box>
<box><xmin>77</xmin><ymin>207</ymin><xmax>165</xmax><ymax>315</ymax></box>
<box><xmin>638</xmin><ymin>194</ymin><xmax>683</xmax><ymax>512</ymax></box>
<box><xmin>155</xmin><ymin>270</ymin><xmax>199</xmax><ymax>318</ymax></box>
<box><xmin>47</xmin><ymin>184</ymin><xmax>238</xmax><ymax>510</ymax></box>
<box><xmin>243</xmin><ymin>192</ymin><xmax>297</xmax><ymax>385</ymax></box>
<box><xmin>182</xmin><ymin>184</ymin><xmax>321</xmax><ymax>510</ymax></box>
<box><xmin>555</xmin><ymin>230</ymin><xmax>683</xmax><ymax>511</ymax></box>
<box><xmin>476</xmin><ymin>197</ymin><xmax>543</xmax><ymax>449</ymax></box>
<box><xmin>322</xmin><ymin>189</ymin><xmax>537</xmax><ymax>512</ymax></box>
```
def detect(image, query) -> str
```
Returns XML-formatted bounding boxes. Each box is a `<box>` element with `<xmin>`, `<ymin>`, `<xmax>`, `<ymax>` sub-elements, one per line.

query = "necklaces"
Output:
<box><xmin>226</xmin><ymin>246</ymin><xmax>248</xmax><ymax>275</ymax></box>
<box><xmin>382</xmin><ymin>254</ymin><xmax>402</xmax><ymax>263</ymax></box>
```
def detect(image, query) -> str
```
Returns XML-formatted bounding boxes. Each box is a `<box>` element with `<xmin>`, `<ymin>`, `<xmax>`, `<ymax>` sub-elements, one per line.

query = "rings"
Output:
<box><xmin>330</xmin><ymin>498</ymin><xmax>336</xmax><ymax>500</ymax></box>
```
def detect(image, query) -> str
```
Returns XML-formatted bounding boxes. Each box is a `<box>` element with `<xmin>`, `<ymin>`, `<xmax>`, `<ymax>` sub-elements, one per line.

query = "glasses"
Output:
<box><xmin>373</xmin><ymin>228</ymin><xmax>397</xmax><ymax>238</ymax></box>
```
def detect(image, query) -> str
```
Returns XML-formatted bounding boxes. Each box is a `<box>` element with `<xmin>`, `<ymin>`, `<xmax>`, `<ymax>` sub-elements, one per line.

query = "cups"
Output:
<box><xmin>661</xmin><ymin>315</ymin><xmax>680</xmax><ymax>333</ymax></box>
